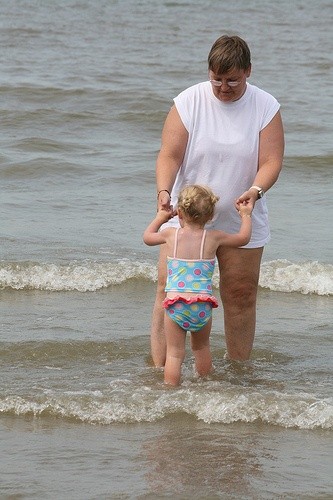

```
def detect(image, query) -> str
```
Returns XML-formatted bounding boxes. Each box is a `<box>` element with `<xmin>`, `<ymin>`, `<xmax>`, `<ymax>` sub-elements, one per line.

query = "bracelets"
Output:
<box><xmin>156</xmin><ymin>190</ymin><xmax>172</xmax><ymax>201</ymax></box>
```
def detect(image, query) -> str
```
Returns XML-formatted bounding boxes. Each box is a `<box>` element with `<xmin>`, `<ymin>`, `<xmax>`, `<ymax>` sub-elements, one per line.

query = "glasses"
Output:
<box><xmin>208</xmin><ymin>68</ymin><xmax>247</xmax><ymax>87</ymax></box>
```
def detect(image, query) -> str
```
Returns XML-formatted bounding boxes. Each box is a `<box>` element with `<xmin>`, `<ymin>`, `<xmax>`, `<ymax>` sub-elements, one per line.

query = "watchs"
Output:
<box><xmin>248</xmin><ymin>186</ymin><xmax>264</xmax><ymax>199</ymax></box>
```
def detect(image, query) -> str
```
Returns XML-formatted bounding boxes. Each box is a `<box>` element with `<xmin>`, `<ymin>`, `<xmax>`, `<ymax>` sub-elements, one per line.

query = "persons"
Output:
<box><xmin>143</xmin><ymin>184</ymin><xmax>254</xmax><ymax>385</ymax></box>
<box><xmin>150</xmin><ymin>33</ymin><xmax>284</xmax><ymax>376</ymax></box>
